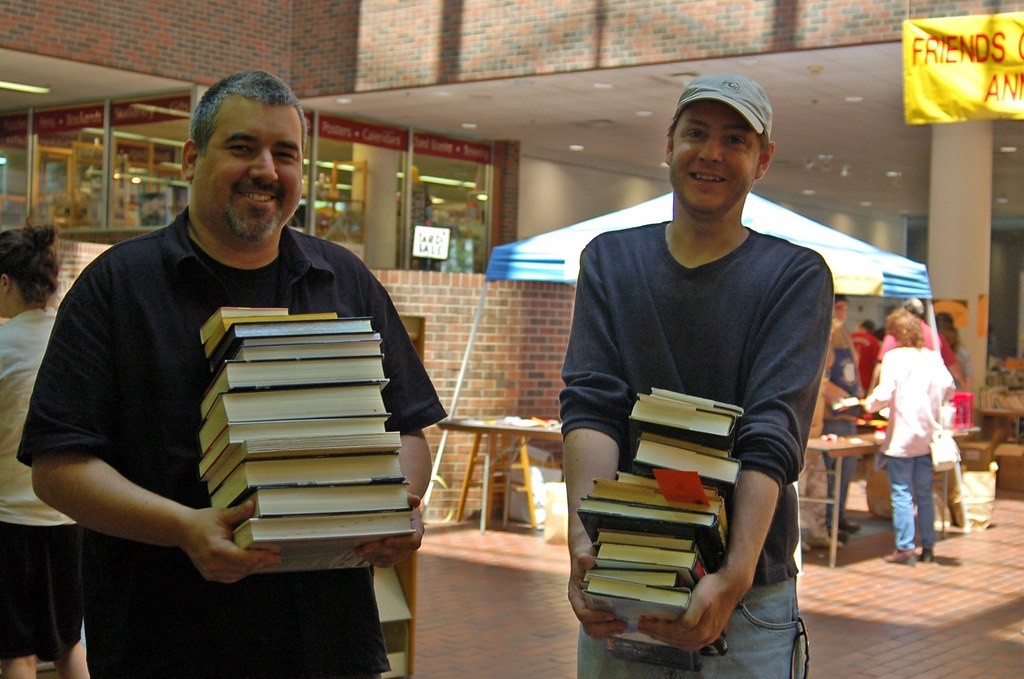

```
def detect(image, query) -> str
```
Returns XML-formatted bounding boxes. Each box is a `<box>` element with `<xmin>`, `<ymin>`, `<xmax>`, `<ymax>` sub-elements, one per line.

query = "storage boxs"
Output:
<box><xmin>960</xmin><ymin>427</ymin><xmax>1024</xmax><ymax>492</ymax></box>
<box><xmin>954</xmin><ymin>393</ymin><xmax>974</xmax><ymax>429</ymax></box>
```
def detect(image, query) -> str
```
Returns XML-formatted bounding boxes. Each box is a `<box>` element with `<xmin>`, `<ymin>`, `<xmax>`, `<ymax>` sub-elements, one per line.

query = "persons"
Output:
<box><xmin>820</xmin><ymin>295</ymin><xmax>865</xmax><ymax>542</ymax></box>
<box><xmin>16</xmin><ymin>69</ymin><xmax>449</xmax><ymax>679</ymax></box>
<box><xmin>0</xmin><ymin>225</ymin><xmax>88</xmax><ymax>679</ymax></box>
<box><xmin>852</xmin><ymin>299</ymin><xmax>974</xmax><ymax>565</ymax></box>
<box><xmin>559</xmin><ymin>73</ymin><xmax>835</xmax><ymax>679</ymax></box>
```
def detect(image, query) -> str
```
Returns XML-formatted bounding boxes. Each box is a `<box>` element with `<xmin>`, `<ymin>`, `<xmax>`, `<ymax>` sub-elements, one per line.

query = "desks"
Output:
<box><xmin>798</xmin><ymin>432</ymin><xmax>949</xmax><ymax>567</ymax></box>
<box><xmin>976</xmin><ymin>407</ymin><xmax>1024</xmax><ymax>417</ymax></box>
<box><xmin>438</xmin><ymin>417</ymin><xmax>562</xmax><ymax>532</ymax></box>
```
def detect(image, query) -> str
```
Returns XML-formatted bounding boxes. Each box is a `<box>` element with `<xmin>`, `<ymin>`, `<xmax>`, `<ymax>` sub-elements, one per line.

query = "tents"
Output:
<box><xmin>420</xmin><ymin>192</ymin><xmax>970</xmax><ymax>567</ymax></box>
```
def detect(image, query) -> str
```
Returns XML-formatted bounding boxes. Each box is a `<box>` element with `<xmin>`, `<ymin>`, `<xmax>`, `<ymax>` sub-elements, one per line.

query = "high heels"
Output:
<box><xmin>919</xmin><ymin>547</ymin><xmax>934</xmax><ymax>562</ymax></box>
<box><xmin>884</xmin><ymin>550</ymin><xmax>915</xmax><ymax>565</ymax></box>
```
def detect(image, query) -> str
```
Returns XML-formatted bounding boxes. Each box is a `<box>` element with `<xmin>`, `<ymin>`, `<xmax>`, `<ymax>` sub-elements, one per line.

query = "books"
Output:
<box><xmin>576</xmin><ymin>388</ymin><xmax>744</xmax><ymax>672</ymax></box>
<box><xmin>200</xmin><ymin>306</ymin><xmax>415</xmax><ymax>571</ymax></box>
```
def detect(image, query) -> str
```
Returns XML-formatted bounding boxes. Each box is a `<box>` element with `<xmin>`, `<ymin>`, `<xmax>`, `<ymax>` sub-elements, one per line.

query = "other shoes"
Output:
<box><xmin>828</xmin><ymin>526</ymin><xmax>848</xmax><ymax>541</ymax></box>
<box><xmin>839</xmin><ymin>518</ymin><xmax>860</xmax><ymax>532</ymax></box>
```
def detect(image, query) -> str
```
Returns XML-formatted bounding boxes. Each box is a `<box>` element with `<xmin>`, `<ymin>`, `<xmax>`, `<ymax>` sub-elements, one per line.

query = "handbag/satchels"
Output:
<box><xmin>949</xmin><ymin>462</ymin><xmax>999</xmax><ymax>531</ymax></box>
<box><xmin>508</xmin><ymin>446</ymin><xmax>570</xmax><ymax>545</ymax></box>
<box><xmin>930</xmin><ymin>428</ymin><xmax>955</xmax><ymax>472</ymax></box>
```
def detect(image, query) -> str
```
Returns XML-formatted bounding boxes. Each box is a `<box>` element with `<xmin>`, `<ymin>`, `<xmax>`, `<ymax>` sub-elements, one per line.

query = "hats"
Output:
<box><xmin>676</xmin><ymin>73</ymin><xmax>773</xmax><ymax>142</ymax></box>
